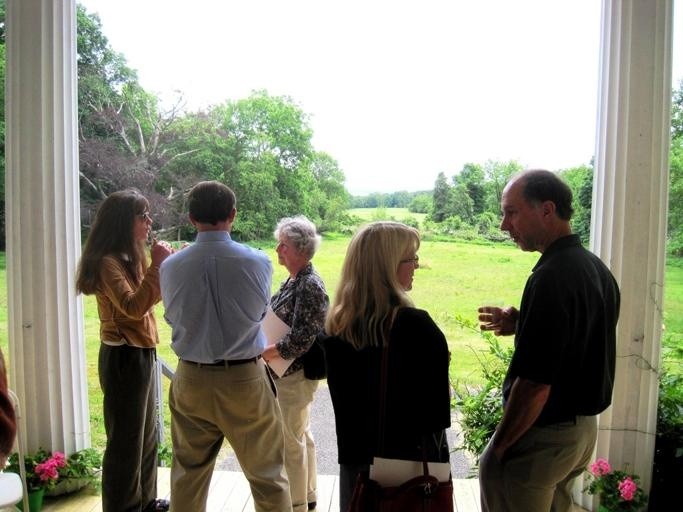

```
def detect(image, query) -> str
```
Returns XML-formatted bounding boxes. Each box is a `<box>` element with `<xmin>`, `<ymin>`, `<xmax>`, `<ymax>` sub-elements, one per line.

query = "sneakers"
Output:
<box><xmin>145</xmin><ymin>498</ymin><xmax>170</xmax><ymax>512</ymax></box>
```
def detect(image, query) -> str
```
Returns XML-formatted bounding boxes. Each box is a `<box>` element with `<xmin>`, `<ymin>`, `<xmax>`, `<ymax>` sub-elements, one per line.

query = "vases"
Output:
<box><xmin>598</xmin><ymin>502</ymin><xmax>637</xmax><ymax>512</ymax></box>
<box><xmin>15</xmin><ymin>483</ymin><xmax>46</xmax><ymax>512</ymax></box>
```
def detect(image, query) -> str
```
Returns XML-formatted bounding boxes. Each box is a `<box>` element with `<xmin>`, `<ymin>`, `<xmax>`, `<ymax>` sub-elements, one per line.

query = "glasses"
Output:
<box><xmin>399</xmin><ymin>256</ymin><xmax>419</xmax><ymax>263</ymax></box>
<box><xmin>136</xmin><ymin>212</ymin><xmax>147</xmax><ymax>220</ymax></box>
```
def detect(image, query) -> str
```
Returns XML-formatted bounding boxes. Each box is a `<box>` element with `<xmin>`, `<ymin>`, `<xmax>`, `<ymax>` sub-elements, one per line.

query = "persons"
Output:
<box><xmin>303</xmin><ymin>221</ymin><xmax>455</xmax><ymax>511</ymax></box>
<box><xmin>259</xmin><ymin>214</ymin><xmax>330</xmax><ymax>511</ymax></box>
<box><xmin>475</xmin><ymin>168</ymin><xmax>621</xmax><ymax>511</ymax></box>
<box><xmin>158</xmin><ymin>181</ymin><xmax>294</xmax><ymax>512</ymax></box>
<box><xmin>75</xmin><ymin>191</ymin><xmax>176</xmax><ymax>511</ymax></box>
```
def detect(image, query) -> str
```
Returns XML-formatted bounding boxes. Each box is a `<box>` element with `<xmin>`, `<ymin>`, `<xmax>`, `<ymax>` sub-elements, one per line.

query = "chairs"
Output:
<box><xmin>0</xmin><ymin>386</ymin><xmax>32</xmax><ymax>511</ymax></box>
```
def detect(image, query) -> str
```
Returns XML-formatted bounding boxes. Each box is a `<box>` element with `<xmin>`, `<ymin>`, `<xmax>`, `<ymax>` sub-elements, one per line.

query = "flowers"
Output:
<box><xmin>584</xmin><ymin>455</ymin><xmax>642</xmax><ymax>507</ymax></box>
<box><xmin>4</xmin><ymin>447</ymin><xmax>103</xmax><ymax>487</ymax></box>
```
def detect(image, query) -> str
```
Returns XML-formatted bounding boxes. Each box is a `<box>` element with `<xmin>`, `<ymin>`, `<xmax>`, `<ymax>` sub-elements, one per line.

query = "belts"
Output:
<box><xmin>187</xmin><ymin>354</ymin><xmax>263</xmax><ymax>366</ymax></box>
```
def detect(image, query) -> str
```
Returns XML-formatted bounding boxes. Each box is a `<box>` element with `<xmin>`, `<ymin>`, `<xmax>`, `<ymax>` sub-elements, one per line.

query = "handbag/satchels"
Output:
<box><xmin>346</xmin><ymin>466</ymin><xmax>454</xmax><ymax>512</ymax></box>
